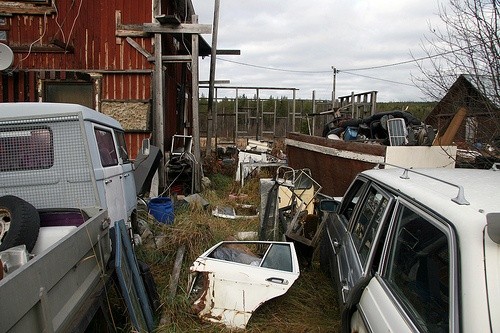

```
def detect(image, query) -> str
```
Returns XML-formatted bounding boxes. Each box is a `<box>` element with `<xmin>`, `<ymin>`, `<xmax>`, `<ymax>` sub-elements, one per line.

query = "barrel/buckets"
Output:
<box><xmin>148</xmin><ymin>197</ymin><xmax>174</xmax><ymax>225</ymax></box>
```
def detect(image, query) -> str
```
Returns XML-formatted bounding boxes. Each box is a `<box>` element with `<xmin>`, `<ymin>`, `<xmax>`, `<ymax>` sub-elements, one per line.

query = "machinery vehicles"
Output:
<box><xmin>0</xmin><ymin>102</ymin><xmax>137</xmax><ymax>333</ymax></box>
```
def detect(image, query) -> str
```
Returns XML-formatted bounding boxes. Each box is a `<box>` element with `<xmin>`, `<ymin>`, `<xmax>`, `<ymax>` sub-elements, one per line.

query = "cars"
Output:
<box><xmin>328</xmin><ymin>162</ymin><xmax>499</xmax><ymax>333</ymax></box>
